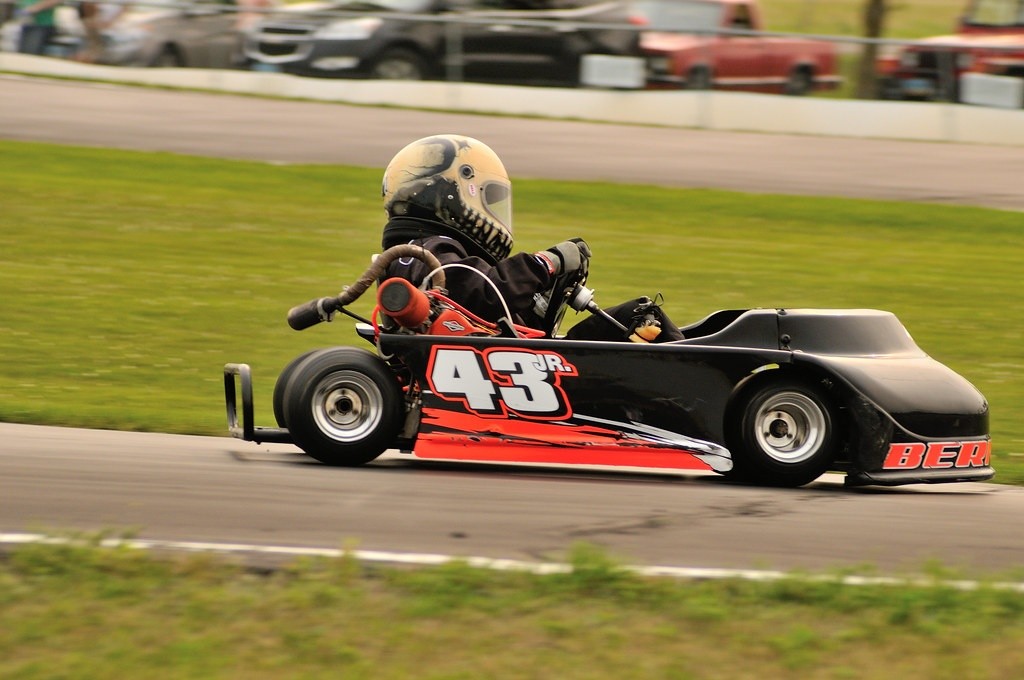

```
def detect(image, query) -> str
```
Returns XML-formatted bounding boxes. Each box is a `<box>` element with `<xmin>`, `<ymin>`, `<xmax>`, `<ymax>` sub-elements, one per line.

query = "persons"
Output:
<box><xmin>376</xmin><ymin>133</ymin><xmax>686</xmax><ymax>345</ymax></box>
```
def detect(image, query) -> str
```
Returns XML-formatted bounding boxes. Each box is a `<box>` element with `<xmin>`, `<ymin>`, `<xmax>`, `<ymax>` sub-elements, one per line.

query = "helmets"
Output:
<box><xmin>381</xmin><ymin>133</ymin><xmax>514</xmax><ymax>263</ymax></box>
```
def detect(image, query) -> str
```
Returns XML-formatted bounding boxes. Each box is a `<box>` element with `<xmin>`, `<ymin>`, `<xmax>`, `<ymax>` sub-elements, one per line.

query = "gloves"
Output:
<box><xmin>532</xmin><ymin>237</ymin><xmax>593</xmax><ymax>277</ymax></box>
<box><xmin>533</xmin><ymin>284</ymin><xmax>574</xmax><ymax>317</ymax></box>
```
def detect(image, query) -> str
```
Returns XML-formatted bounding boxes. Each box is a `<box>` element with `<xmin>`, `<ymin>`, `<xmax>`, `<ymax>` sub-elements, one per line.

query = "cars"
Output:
<box><xmin>624</xmin><ymin>1</ymin><xmax>843</xmax><ymax>95</ymax></box>
<box><xmin>897</xmin><ymin>0</ymin><xmax>1024</xmax><ymax>80</ymax></box>
<box><xmin>245</xmin><ymin>0</ymin><xmax>645</xmax><ymax>88</ymax></box>
<box><xmin>98</xmin><ymin>0</ymin><xmax>274</xmax><ymax>69</ymax></box>
